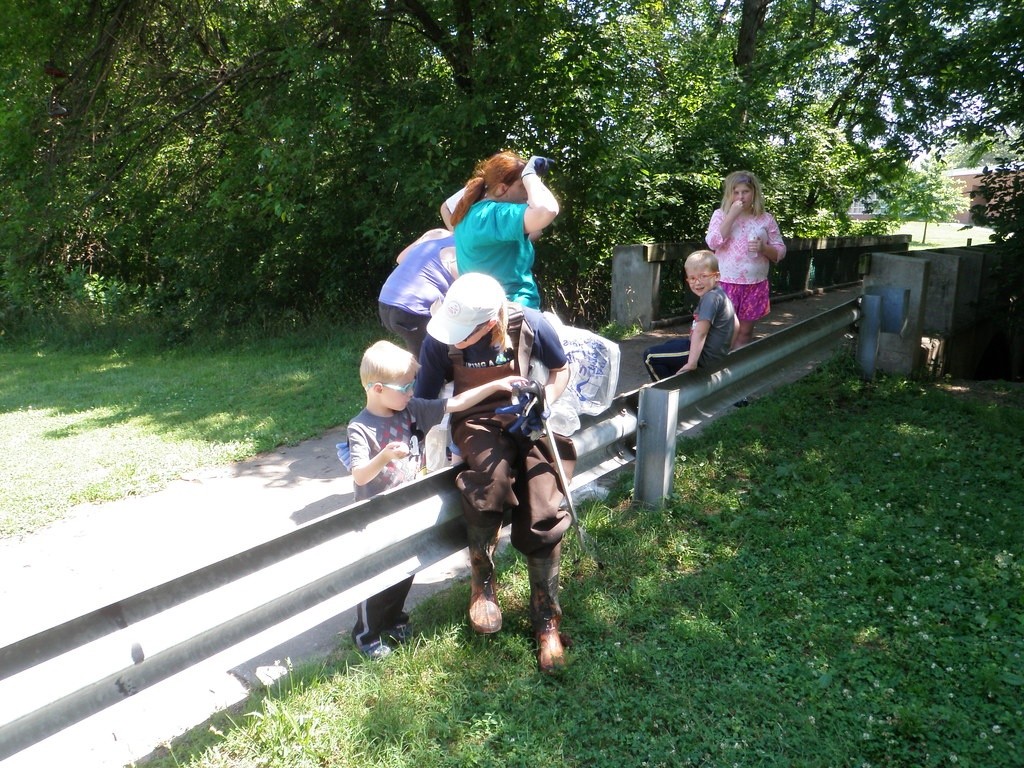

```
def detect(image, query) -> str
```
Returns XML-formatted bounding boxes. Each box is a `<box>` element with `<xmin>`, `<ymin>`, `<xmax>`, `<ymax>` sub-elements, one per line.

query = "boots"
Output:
<box><xmin>526</xmin><ymin>552</ymin><xmax>564</xmax><ymax>675</ymax></box>
<box><xmin>464</xmin><ymin>517</ymin><xmax>502</xmax><ymax>633</ymax></box>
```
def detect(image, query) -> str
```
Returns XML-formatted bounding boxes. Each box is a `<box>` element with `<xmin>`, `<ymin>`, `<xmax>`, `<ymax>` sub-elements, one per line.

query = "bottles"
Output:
<box><xmin>747</xmin><ymin>226</ymin><xmax>759</xmax><ymax>257</ymax></box>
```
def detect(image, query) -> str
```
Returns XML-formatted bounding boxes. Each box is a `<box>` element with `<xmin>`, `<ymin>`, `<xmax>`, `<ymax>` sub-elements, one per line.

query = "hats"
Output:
<box><xmin>426</xmin><ymin>273</ymin><xmax>504</xmax><ymax>345</ymax></box>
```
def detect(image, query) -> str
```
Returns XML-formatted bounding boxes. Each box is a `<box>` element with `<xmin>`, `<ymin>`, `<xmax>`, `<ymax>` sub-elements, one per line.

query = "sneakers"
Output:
<box><xmin>386</xmin><ymin>622</ymin><xmax>410</xmax><ymax>643</ymax></box>
<box><xmin>355</xmin><ymin>639</ymin><xmax>390</xmax><ymax>659</ymax></box>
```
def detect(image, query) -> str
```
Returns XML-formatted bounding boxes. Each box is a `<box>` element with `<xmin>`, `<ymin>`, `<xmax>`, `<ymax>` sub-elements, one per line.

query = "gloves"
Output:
<box><xmin>520</xmin><ymin>155</ymin><xmax>555</xmax><ymax>178</ymax></box>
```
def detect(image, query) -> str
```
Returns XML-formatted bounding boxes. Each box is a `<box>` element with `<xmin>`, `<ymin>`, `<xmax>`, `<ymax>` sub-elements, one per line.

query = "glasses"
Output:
<box><xmin>686</xmin><ymin>272</ymin><xmax>716</xmax><ymax>285</ymax></box>
<box><xmin>367</xmin><ymin>376</ymin><xmax>416</xmax><ymax>394</ymax></box>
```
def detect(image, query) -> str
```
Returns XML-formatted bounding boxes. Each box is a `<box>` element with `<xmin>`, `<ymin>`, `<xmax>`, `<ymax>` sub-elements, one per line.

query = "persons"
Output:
<box><xmin>411</xmin><ymin>273</ymin><xmax>577</xmax><ymax>672</ymax></box>
<box><xmin>376</xmin><ymin>227</ymin><xmax>459</xmax><ymax>361</ymax></box>
<box><xmin>643</xmin><ymin>249</ymin><xmax>740</xmax><ymax>381</ymax></box>
<box><xmin>705</xmin><ymin>170</ymin><xmax>787</xmax><ymax>408</ymax></box>
<box><xmin>440</xmin><ymin>151</ymin><xmax>500</xmax><ymax>232</ymax></box>
<box><xmin>347</xmin><ymin>338</ymin><xmax>529</xmax><ymax>660</ymax></box>
<box><xmin>453</xmin><ymin>152</ymin><xmax>561</xmax><ymax>310</ymax></box>
